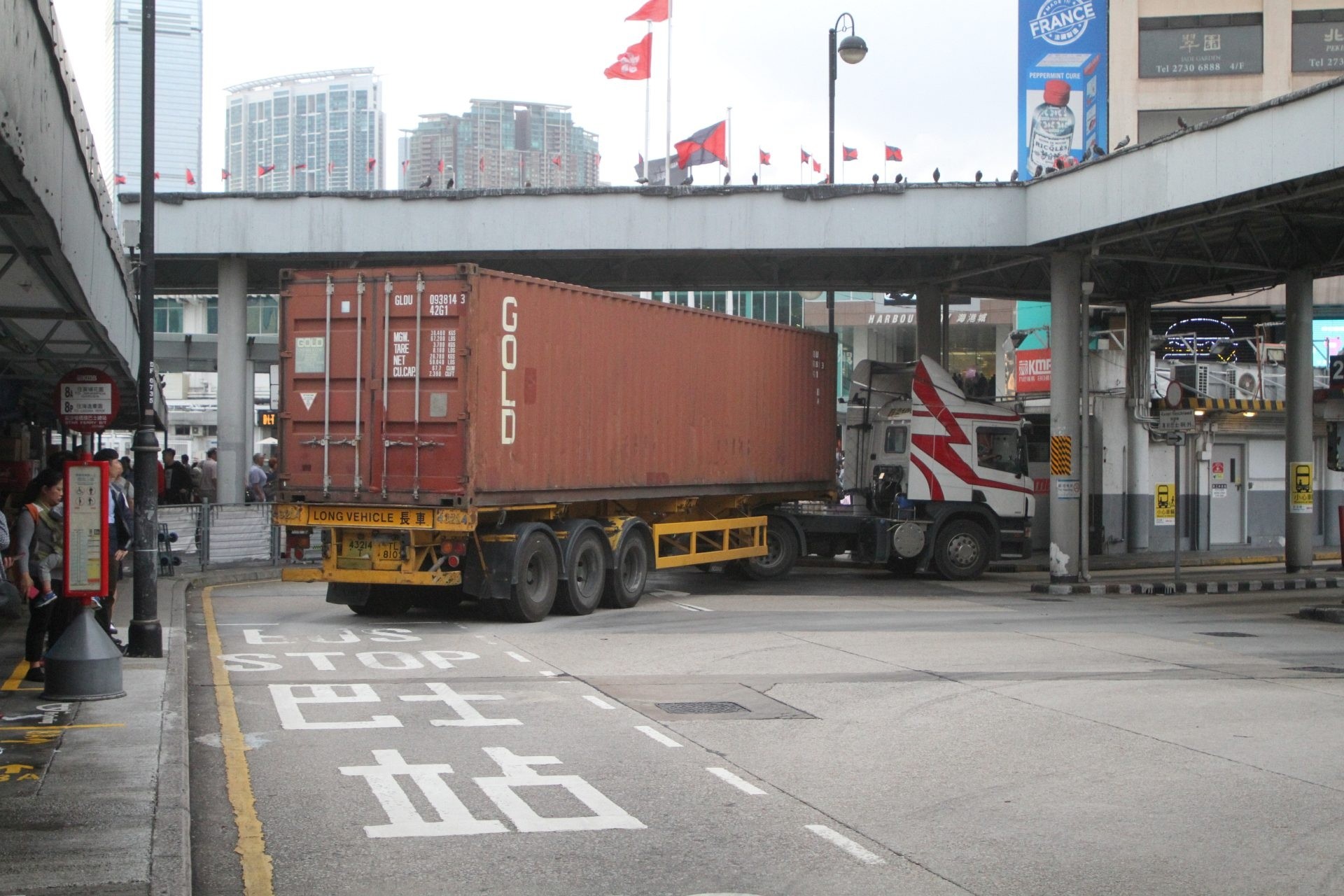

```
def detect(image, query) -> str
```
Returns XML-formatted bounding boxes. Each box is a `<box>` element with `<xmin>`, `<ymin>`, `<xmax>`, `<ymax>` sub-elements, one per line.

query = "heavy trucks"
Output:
<box><xmin>271</xmin><ymin>263</ymin><xmax>1038</xmax><ymax>624</ymax></box>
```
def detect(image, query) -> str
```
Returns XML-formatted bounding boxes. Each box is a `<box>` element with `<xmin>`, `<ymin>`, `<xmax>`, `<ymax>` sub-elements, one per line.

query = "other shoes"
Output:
<box><xmin>117</xmin><ymin>644</ymin><xmax>129</xmax><ymax>655</ymax></box>
<box><xmin>112</xmin><ymin>637</ymin><xmax>121</xmax><ymax>644</ymax></box>
<box><xmin>27</xmin><ymin>667</ymin><xmax>45</xmax><ymax>682</ymax></box>
<box><xmin>110</xmin><ymin>622</ymin><xmax>117</xmax><ymax>633</ymax></box>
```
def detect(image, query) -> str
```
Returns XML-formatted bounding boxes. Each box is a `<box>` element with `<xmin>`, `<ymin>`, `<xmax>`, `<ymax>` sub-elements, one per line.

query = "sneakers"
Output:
<box><xmin>90</xmin><ymin>596</ymin><xmax>102</xmax><ymax>610</ymax></box>
<box><xmin>34</xmin><ymin>591</ymin><xmax>58</xmax><ymax>609</ymax></box>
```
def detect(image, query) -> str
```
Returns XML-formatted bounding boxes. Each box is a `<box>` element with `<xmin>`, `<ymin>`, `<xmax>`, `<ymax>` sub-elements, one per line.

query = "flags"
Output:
<box><xmin>758</xmin><ymin>148</ymin><xmax>771</xmax><ymax>166</ymax></box>
<box><xmin>720</xmin><ymin>155</ymin><xmax>727</xmax><ymax>168</ymax></box>
<box><xmin>813</xmin><ymin>159</ymin><xmax>821</xmax><ymax>172</ymax></box>
<box><xmin>625</xmin><ymin>0</ymin><xmax>672</xmax><ymax>22</ymax></box>
<box><xmin>114</xmin><ymin>152</ymin><xmax>647</xmax><ymax>188</ymax></box>
<box><xmin>842</xmin><ymin>146</ymin><xmax>857</xmax><ymax>161</ymax></box>
<box><xmin>604</xmin><ymin>32</ymin><xmax>653</xmax><ymax>80</ymax></box>
<box><xmin>885</xmin><ymin>146</ymin><xmax>902</xmax><ymax>162</ymax></box>
<box><xmin>801</xmin><ymin>151</ymin><xmax>811</xmax><ymax>164</ymax></box>
<box><xmin>674</xmin><ymin>120</ymin><xmax>728</xmax><ymax>169</ymax></box>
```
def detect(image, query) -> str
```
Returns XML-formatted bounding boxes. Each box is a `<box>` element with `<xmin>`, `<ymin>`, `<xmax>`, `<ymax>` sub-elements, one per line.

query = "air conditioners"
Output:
<box><xmin>1170</xmin><ymin>364</ymin><xmax>1208</xmax><ymax>398</ymax></box>
<box><xmin>1225</xmin><ymin>368</ymin><xmax>1265</xmax><ymax>400</ymax></box>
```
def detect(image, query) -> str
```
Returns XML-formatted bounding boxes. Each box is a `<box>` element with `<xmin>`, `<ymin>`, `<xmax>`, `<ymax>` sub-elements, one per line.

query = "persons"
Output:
<box><xmin>0</xmin><ymin>444</ymin><xmax>277</xmax><ymax>681</ymax></box>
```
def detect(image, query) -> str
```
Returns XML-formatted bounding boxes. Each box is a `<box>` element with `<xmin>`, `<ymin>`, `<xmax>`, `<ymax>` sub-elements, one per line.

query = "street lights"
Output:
<box><xmin>829</xmin><ymin>10</ymin><xmax>870</xmax><ymax>333</ymax></box>
